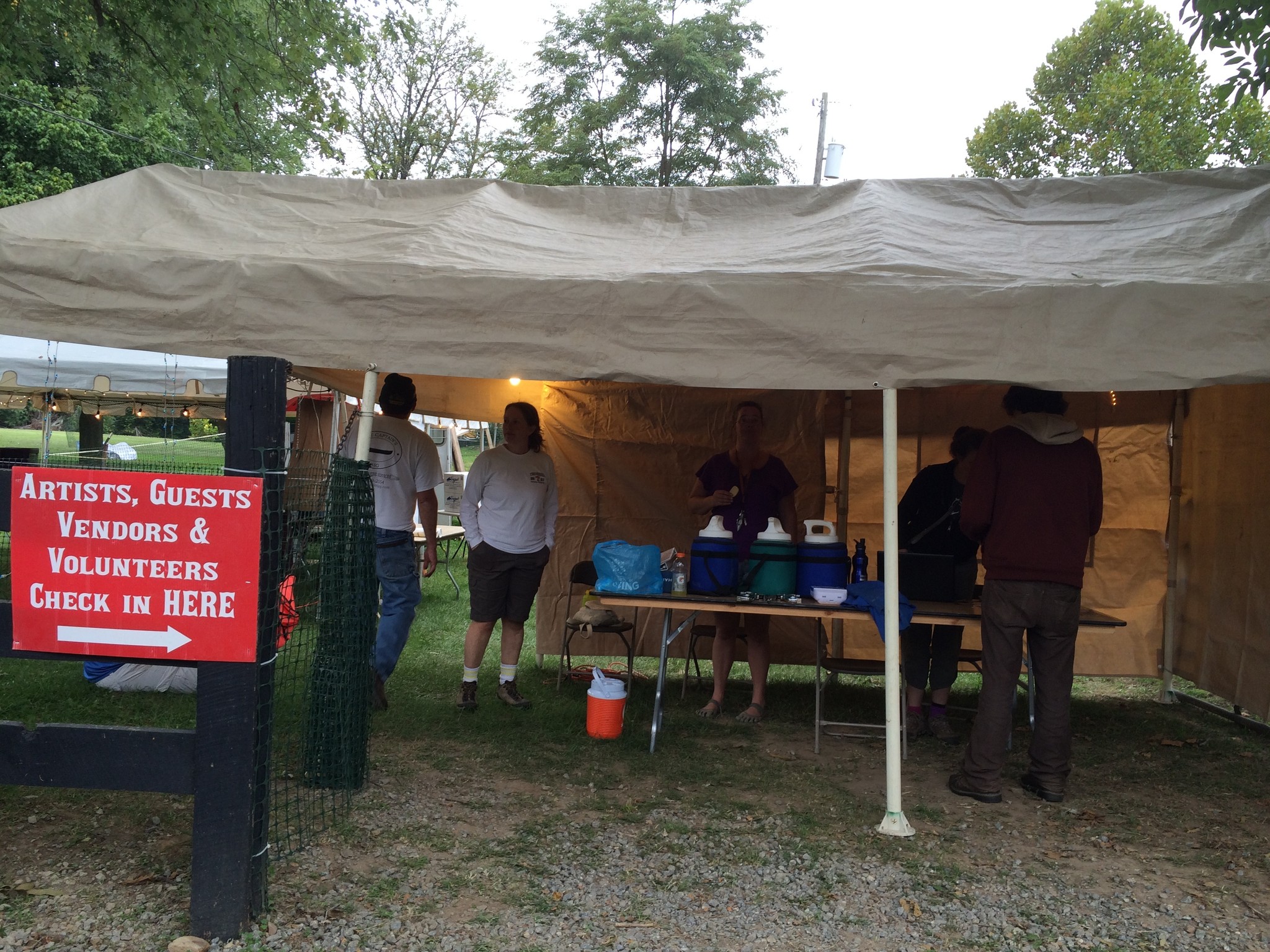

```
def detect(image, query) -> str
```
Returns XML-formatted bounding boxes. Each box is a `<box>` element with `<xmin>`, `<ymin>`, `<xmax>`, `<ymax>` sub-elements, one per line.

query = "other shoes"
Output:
<box><xmin>372</xmin><ymin>674</ymin><xmax>388</xmax><ymax>711</ymax></box>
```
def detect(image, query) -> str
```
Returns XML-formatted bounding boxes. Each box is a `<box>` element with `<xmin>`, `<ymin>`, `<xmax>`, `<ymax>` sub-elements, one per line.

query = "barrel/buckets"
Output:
<box><xmin>796</xmin><ymin>519</ymin><xmax>849</xmax><ymax>598</ymax></box>
<box><xmin>746</xmin><ymin>517</ymin><xmax>796</xmax><ymax>596</ymax></box>
<box><xmin>586</xmin><ymin>666</ymin><xmax>628</xmax><ymax>739</ymax></box>
<box><xmin>688</xmin><ymin>516</ymin><xmax>741</xmax><ymax>596</ymax></box>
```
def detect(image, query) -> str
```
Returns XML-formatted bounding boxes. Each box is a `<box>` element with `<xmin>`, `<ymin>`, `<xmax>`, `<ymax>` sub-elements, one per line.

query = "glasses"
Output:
<box><xmin>736</xmin><ymin>415</ymin><xmax>763</xmax><ymax>425</ymax></box>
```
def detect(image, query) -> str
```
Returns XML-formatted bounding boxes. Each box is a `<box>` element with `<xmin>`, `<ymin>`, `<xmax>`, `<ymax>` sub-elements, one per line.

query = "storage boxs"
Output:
<box><xmin>443</xmin><ymin>492</ymin><xmax>463</xmax><ymax>513</ymax></box>
<box><xmin>442</xmin><ymin>470</ymin><xmax>470</xmax><ymax>494</ymax></box>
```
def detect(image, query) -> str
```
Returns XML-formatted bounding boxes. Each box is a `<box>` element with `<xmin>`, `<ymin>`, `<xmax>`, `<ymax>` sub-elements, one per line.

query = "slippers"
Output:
<box><xmin>736</xmin><ymin>702</ymin><xmax>766</xmax><ymax>722</ymax></box>
<box><xmin>695</xmin><ymin>697</ymin><xmax>727</xmax><ymax>718</ymax></box>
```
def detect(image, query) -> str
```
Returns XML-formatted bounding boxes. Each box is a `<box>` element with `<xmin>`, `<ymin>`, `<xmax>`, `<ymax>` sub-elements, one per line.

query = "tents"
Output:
<box><xmin>0</xmin><ymin>333</ymin><xmax>495</xmax><ymax>627</ymax></box>
<box><xmin>0</xmin><ymin>160</ymin><xmax>1270</xmax><ymax>834</ymax></box>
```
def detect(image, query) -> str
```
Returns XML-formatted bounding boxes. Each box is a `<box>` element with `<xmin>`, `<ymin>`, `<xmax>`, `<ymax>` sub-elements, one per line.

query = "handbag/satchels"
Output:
<box><xmin>660</xmin><ymin>547</ymin><xmax>677</xmax><ymax>571</ymax></box>
<box><xmin>567</xmin><ymin>599</ymin><xmax>624</xmax><ymax>639</ymax></box>
<box><xmin>591</xmin><ymin>540</ymin><xmax>663</xmax><ymax>594</ymax></box>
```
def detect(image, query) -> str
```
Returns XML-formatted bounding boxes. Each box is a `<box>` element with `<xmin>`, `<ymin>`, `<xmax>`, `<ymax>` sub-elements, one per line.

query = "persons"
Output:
<box><xmin>693</xmin><ymin>401</ymin><xmax>801</xmax><ymax>723</ymax></box>
<box><xmin>459</xmin><ymin>398</ymin><xmax>558</xmax><ymax>715</ymax></box>
<box><xmin>338</xmin><ymin>371</ymin><xmax>443</xmax><ymax>709</ymax></box>
<box><xmin>949</xmin><ymin>383</ymin><xmax>1104</xmax><ymax>801</ymax></box>
<box><xmin>898</xmin><ymin>427</ymin><xmax>988</xmax><ymax>716</ymax></box>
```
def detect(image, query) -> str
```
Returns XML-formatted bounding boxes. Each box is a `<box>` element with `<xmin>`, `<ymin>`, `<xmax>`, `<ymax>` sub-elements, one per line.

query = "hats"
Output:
<box><xmin>380</xmin><ymin>373</ymin><xmax>416</xmax><ymax>414</ymax></box>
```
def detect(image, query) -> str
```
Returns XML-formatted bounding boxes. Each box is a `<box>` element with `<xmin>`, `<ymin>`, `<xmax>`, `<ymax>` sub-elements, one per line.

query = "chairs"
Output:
<box><xmin>948</xmin><ymin>645</ymin><xmax>985</xmax><ymax>717</ymax></box>
<box><xmin>811</xmin><ymin>616</ymin><xmax>913</xmax><ymax>761</ymax></box>
<box><xmin>554</xmin><ymin>559</ymin><xmax>640</xmax><ymax>702</ymax></box>
<box><xmin>679</xmin><ymin>609</ymin><xmax>748</xmax><ymax>702</ymax></box>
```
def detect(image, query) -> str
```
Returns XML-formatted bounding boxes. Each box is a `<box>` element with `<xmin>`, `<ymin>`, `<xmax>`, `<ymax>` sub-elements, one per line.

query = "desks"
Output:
<box><xmin>598</xmin><ymin>587</ymin><xmax>1129</xmax><ymax>758</ymax></box>
<box><xmin>437</xmin><ymin>509</ymin><xmax>468</xmax><ymax>561</ymax></box>
<box><xmin>411</xmin><ymin>522</ymin><xmax>467</xmax><ymax>601</ymax></box>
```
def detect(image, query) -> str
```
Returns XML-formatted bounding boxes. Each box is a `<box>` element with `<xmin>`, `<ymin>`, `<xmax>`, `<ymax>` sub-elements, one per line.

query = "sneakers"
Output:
<box><xmin>903</xmin><ymin>710</ymin><xmax>928</xmax><ymax>737</ymax></box>
<box><xmin>456</xmin><ymin>680</ymin><xmax>480</xmax><ymax>708</ymax></box>
<box><xmin>498</xmin><ymin>679</ymin><xmax>530</xmax><ymax>708</ymax></box>
<box><xmin>926</xmin><ymin>714</ymin><xmax>957</xmax><ymax>739</ymax></box>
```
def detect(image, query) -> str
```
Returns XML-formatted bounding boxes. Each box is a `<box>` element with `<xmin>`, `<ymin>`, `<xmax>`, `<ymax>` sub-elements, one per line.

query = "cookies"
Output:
<box><xmin>729</xmin><ymin>486</ymin><xmax>739</xmax><ymax>497</ymax></box>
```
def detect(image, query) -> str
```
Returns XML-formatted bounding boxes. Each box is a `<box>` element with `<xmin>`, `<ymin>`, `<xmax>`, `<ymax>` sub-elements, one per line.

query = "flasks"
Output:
<box><xmin>851</xmin><ymin>538</ymin><xmax>869</xmax><ymax>582</ymax></box>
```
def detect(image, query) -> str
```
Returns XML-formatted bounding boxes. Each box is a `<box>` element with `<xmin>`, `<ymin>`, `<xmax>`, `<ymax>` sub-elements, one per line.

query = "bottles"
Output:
<box><xmin>670</xmin><ymin>552</ymin><xmax>687</xmax><ymax>597</ymax></box>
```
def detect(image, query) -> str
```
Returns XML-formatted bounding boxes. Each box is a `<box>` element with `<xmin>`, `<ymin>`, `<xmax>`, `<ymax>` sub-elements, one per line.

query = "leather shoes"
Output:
<box><xmin>1021</xmin><ymin>773</ymin><xmax>1064</xmax><ymax>802</ymax></box>
<box><xmin>949</xmin><ymin>774</ymin><xmax>1002</xmax><ymax>802</ymax></box>
